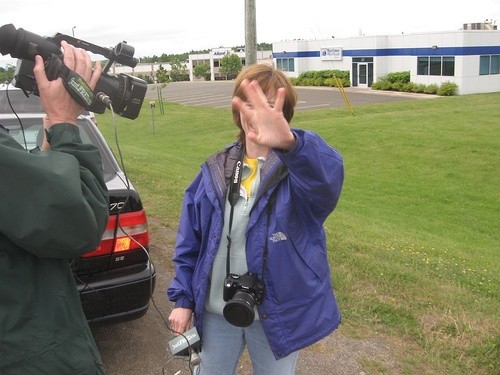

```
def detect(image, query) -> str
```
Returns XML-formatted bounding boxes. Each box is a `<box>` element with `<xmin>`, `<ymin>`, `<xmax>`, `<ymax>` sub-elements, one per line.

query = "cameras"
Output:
<box><xmin>223</xmin><ymin>272</ymin><xmax>265</xmax><ymax>327</ymax></box>
<box><xmin>0</xmin><ymin>24</ymin><xmax>148</xmax><ymax>120</ymax></box>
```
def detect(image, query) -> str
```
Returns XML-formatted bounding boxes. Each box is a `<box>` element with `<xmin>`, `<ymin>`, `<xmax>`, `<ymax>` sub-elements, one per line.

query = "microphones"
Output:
<box><xmin>182</xmin><ymin>328</ymin><xmax>200</xmax><ymax>365</ymax></box>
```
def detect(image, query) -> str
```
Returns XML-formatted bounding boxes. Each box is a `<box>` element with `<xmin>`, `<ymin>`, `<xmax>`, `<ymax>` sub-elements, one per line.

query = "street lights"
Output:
<box><xmin>72</xmin><ymin>26</ymin><xmax>76</xmax><ymax>37</ymax></box>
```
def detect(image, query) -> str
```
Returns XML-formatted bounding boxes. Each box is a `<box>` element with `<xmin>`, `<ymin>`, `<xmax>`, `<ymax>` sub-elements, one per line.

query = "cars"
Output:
<box><xmin>1</xmin><ymin>112</ymin><xmax>157</xmax><ymax>326</ymax></box>
<box><xmin>1</xmin><ymin>82</ymin><xmax>99</xmax><ymax>130</ymax></box>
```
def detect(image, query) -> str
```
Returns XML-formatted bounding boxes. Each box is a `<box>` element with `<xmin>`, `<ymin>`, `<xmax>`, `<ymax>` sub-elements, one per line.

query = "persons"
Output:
<box><xmin>0</xmin><ymin>39</ymin><xmax>111</xmax><ymax>374</ymax></box>
<box><xmin>165</xmin><ymin>63</ymin><xmax>346</xmax><ymax>375</ymax></box>
